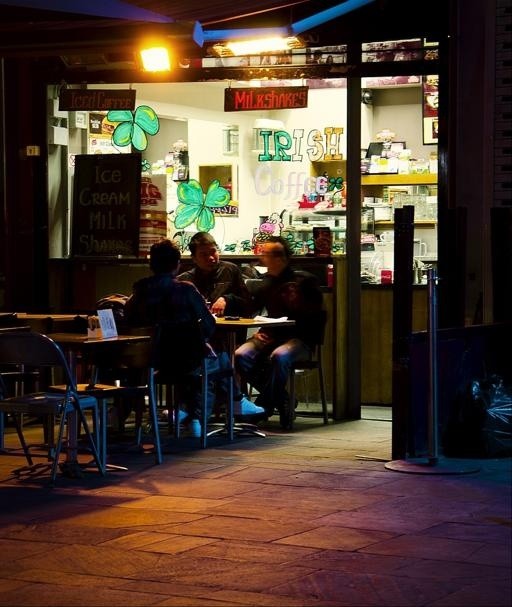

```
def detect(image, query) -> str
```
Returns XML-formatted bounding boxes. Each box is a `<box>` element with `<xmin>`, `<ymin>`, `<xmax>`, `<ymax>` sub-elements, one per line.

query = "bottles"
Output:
<box><xmin>166</xmin><ymin>140</ymin><xmax>189</xmax><ymax>181</ymax></box>
<box><xmin>302</xmin><ymin>173</ymin><xmax>347</xmax><ymax>211</ymax></box>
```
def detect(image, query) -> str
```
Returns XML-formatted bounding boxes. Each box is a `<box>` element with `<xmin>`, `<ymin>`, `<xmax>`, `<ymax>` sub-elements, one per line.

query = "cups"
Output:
<box><xmin>359</xmin><ymin>132</ymin><xmax>438</xmax><ymax>284</ymax></box>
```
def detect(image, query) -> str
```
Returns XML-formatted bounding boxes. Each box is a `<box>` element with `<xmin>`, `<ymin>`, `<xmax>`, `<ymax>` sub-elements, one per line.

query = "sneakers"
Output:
<box><xmin>163</xmin><ymin>409</ymin><xmax>188</xmax><ymax>423</ymax></box>
<box><xmin>279</xmin><ymin>395</ymin><xmax>298</xmax><ymax>428</ymax></box>
<box><xmin>233</xmin><ymin>397</ymin><xmax>264</xmax><ymax>415</ymax></box>
<box><xmin>190</xmin><ymin>418</ymin><xmax>202</xmax><ymax>438</ymax></box>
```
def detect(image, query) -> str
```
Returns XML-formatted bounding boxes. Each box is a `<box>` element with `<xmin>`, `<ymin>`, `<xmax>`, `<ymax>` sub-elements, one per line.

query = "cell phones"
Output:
<box><xmin>225</xmin><ymin>315</ymin><xmax>240</xmax><ymax>320</ymax></box>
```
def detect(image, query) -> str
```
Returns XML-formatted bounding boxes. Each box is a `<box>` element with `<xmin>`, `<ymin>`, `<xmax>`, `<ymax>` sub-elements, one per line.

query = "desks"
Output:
<box><xmin>173</xmin><ymin>314</ymin><xmax>296</xmax><ymax>443</ymax></box>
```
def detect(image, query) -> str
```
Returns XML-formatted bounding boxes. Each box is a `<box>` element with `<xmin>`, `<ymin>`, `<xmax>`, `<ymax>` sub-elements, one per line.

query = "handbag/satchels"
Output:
<box><xmin>96</xmin><ymin>293</ymin><xmax>129</xmax><ymax>318</ymax></box>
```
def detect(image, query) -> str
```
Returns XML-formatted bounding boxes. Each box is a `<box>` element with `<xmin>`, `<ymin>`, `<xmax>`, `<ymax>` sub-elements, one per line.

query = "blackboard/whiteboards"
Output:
<box><xmin>70</xmin><ymin>153</ymin><xmax>141</xmax><ymax>256</ymax></box>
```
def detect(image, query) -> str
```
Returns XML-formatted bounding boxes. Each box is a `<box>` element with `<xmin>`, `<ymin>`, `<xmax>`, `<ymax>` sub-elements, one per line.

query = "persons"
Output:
<box><xmin>214</xmin><ymin>236</ymin><xmax>327</xmax><ymax>428</ymax></box>
<box><xmin>174</xmin><ymin>231</ymin><xmax>254</xmax><ymax>421</ymax></box>
<box><xmin>122</xmin><ymin>239</ymin><xmax>217</xmax><ymax>434</ymax></box>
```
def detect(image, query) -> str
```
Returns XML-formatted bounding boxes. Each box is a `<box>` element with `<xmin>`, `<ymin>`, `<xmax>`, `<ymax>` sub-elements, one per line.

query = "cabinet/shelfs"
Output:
<box><xmin>280</xmin><ymin>207</ymin><xmax>376</xmax><ymax>251</ymax></box>
<box><xmin>361</xmin><ymin>173</ymin><xmax>438</xmax><ymax>265</ymax></box>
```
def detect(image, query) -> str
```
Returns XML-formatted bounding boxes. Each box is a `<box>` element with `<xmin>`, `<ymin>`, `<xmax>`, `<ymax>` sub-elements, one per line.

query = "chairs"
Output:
<box><xmin>247</xmin><ymin>309</ymin><xmax>329</xmax><ymax>424</ymax></box>
